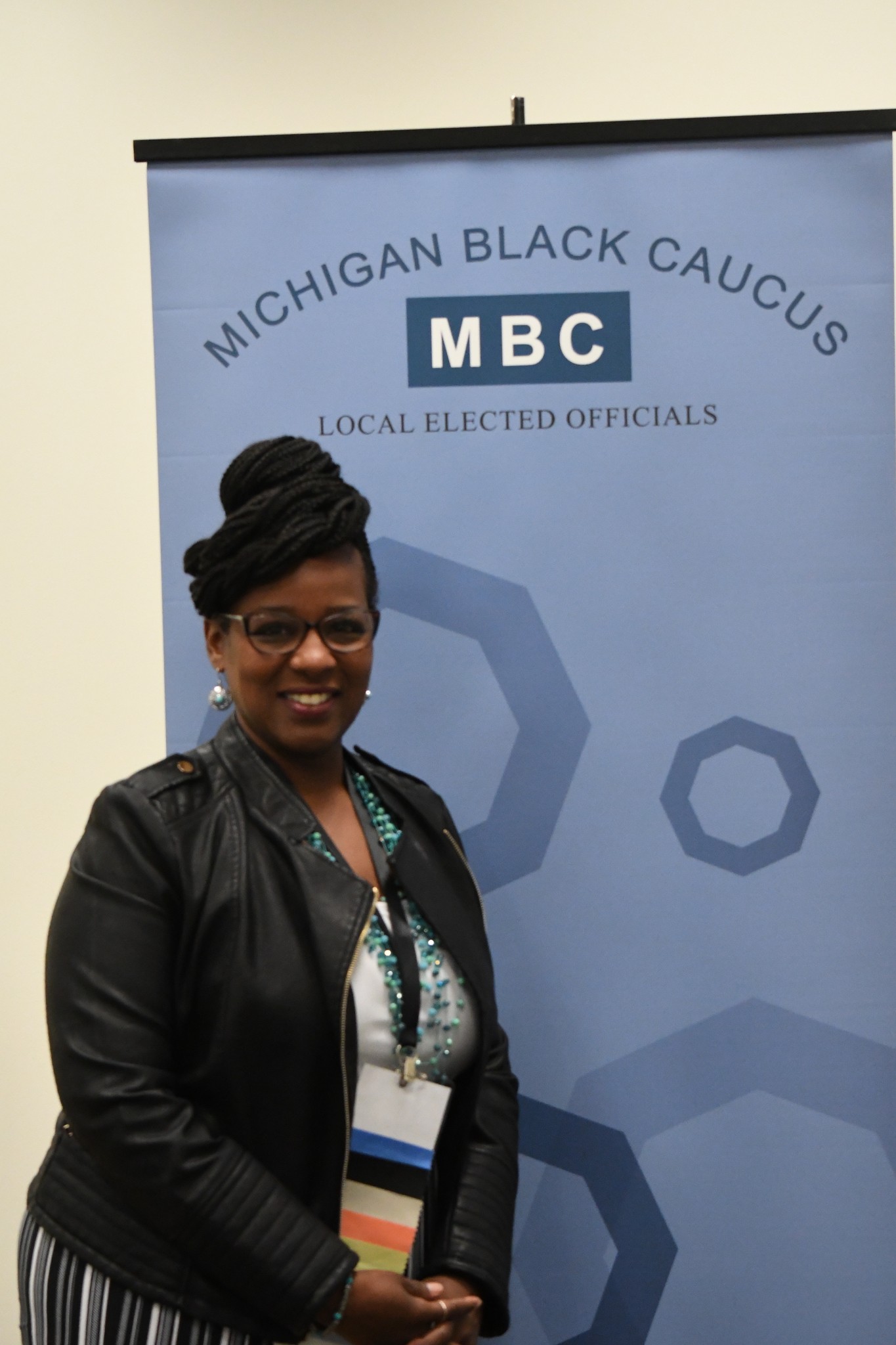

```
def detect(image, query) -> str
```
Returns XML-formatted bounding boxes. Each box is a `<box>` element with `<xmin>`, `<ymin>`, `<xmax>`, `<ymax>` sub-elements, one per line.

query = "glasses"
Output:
<box><xmin>219</xmin><ymin>604</ymin><xmax>373</xmax><ymax>656</ymax></box>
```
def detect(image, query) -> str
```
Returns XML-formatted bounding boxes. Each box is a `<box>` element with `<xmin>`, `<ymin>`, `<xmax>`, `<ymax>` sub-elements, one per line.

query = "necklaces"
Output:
<box><xmin>295</xmin><ymin>755</ymin><xmax>465</xmax><ymax>1087</ymax></box>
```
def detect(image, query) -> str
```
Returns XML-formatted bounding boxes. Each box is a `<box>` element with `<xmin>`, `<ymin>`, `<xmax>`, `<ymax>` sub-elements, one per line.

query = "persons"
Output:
<box><xmin>17</xmin><ymin>437</ymin><xmax>518</xmax><ymax>1345</ymax></box>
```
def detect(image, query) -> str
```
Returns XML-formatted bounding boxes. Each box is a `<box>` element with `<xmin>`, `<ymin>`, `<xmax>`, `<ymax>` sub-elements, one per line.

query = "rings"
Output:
<box><xmin>438</xmin><ymin>1299</ymin><xmax>449</xmax><ymax>1323</ymax></box>
